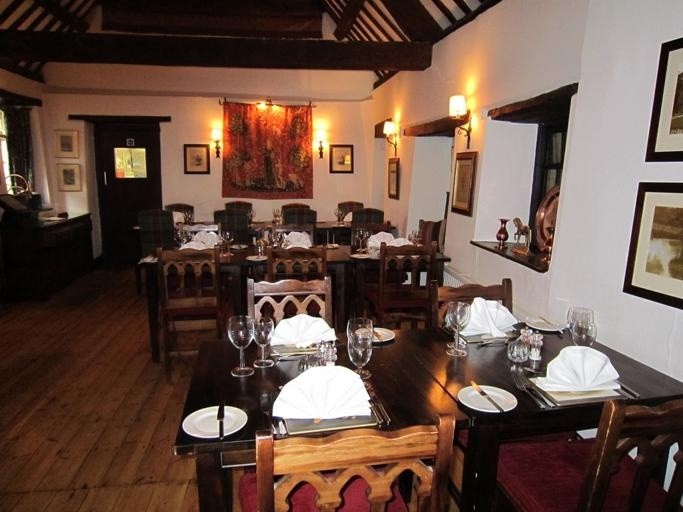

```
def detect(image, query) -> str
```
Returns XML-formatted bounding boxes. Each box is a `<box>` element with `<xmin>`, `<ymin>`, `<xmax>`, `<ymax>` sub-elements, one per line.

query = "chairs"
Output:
<box><xmin>239</xmin><ymin>414</ymin><xmax>459</xmax><ymax>512</ymax></box>
<box><xmin>430</xmin><ymin>279</ymin><xmax>516</xmax><ymax>340</ymax></box>
<box><xmin>458</xmin><ymin>397</ymin><xmax>683</xmax><ymax>512</ymax></box>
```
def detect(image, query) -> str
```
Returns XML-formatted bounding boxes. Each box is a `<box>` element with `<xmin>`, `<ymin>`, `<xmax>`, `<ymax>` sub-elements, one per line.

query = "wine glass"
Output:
<box><xmin>350</xmin><ymin>225</ymin><xmax>425</xmax><ymax>260</ymax></box>
<box><xmin>227</xmin><ymin>314</ymin><xmax>257</xmax><ymax>378</ymax></box>
<box><xmin>297</xmin><ymin>317</ymin><xmax>375</xmax><ymax>381</ymax></box>
<box><xmin>173</xmin><ymin>208</ymin><xmax>289</xmax><ymax>260</ymax></box>
<box><xmin>250</xmin><ymin>316</ymin><xmax>275</xmax><ymax>368</ymax></box>
<box><xmin>333</xmin><ymin>207</ymin><xmax>346</xmax><ymax>225</ymax></box>
<box><xmin>444</xmin><ymin>301</ymin><xmax>470</xmax><ymax>356</ymax></box>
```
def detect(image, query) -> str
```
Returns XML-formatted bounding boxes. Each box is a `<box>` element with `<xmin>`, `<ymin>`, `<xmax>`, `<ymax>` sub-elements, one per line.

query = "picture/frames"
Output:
<box><xmin>388</xmin><ymin>157</ymin><xmax>401</xmax><ymax>200</ymax></box>
<box><xmin>644</xmin><ymin>37</ymin><xmax>683</xmax><ymax>164</ymax></box>
<box><xmin>55</xmin><ymin>162</ymin><xmax>81</xmax><ymax>192</ymax></box>
<box><xmin>622</xmin><ymin>182</ymin><xmax>682</xmax><ymax>312</ymax></box>
<box><xmin>184</xmin><ymin>143</ymin><xmax>212</xmax><ymax>175</ymax></box>
<box><xmin>450</xmin><ymin>151</ymin><xmax>477</xmax><ymax>218</ymax></box>
<box><xmin>53</xmin><ymin>130</ymin><xmax>78</xmax><ymax>159</ymax></box>
<box><xmin>330</xmin><ymin>145</ymin><xmax>355</xmax><ymax>174</ymax></box>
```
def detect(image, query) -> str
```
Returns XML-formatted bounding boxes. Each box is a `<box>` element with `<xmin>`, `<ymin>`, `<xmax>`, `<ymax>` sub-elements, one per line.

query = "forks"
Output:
<box><xmin>512</xmin><ymin>373</ymin><xmax>547</xmax><ymax>410</ymax></box>
<box><xmin>269</xmin><ymin>392</ymin><xmax>289</xmax><ymax>436</ymax></box>
<box><xmin>519</xmin><ymin>371</ymin><xmax>556</xmax><ymax>408</ymax></box>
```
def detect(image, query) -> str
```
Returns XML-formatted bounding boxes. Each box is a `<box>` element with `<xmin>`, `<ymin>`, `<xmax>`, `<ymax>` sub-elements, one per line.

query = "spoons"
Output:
<box><xmin>258</xmin><ymin>393</ymin><xmax>279</xmax><ymax>435</ymax></box>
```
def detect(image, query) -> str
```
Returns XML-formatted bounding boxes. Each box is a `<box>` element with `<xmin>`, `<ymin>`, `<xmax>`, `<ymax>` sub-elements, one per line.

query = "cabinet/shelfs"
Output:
<box><xmin>11</xmin><ymin>212</ymin><xmax>92</xmax><ymax>302</ymax></box>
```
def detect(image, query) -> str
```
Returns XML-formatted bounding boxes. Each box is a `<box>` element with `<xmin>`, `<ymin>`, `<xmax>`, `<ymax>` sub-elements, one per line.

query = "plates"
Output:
<box><xmin>181</xmin><ymin>406</ymin><xmax>249</xmax><ymax>439</ymax></box>
<box><xmin>354</xmin><ymin>326</ymin><xmax>397</xmax><ymax>343</ymax></box>
<box><xmin>524</xmin><ymin>317</ymin><xmax>569</xmax><ymax>332</ymax></box>
<box><xmin>316</xmin><ymin>220</ymin><xmax>326</xmax><ymax>223</ymax></box>
<box><xmin>456</xmin><ymin>384</ymin><xmax>520</xmax><ymax>415</ymax></box>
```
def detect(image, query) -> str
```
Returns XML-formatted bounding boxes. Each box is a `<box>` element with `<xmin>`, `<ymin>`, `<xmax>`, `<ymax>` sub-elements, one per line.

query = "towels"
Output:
<box><xmin>460</xmin><ymin>295</ymin><xmax>520</xmax><ymax>337</ymax></box>
<box><xmin>272</xmin><ymin>365</ymin><xmax>373</xmax><ymax>419</ymax></box>
<box><xmin>535</xmin><ymin>345</ymin><xmax>622</xmax><ymax>393</ymax></box>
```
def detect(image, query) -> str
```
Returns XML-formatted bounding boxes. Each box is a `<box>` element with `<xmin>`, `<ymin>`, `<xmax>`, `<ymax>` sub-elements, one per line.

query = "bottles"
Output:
<box><xmin>542</xmin><ymin>226</ymin><xmax>555</xmax><ymax>263</ymax></box>
<box><xmin>493</xmin><ymin>218</ymin><xmax>513</xmax><ymax>250</ymax></box>
<box><xmin>520</xmin><ymin>325</ymin><xmax>545</xmax><ymax>361</ymax></box>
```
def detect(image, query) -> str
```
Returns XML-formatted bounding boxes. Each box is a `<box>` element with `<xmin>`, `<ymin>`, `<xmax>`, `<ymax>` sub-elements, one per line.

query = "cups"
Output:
<box><xmin>507</xmin><ymin>340</ymin><xmax>530</xmax><ymax>364</ymax></box>
<box><xmin>567</xmin><ymin>306</ymin><xmax>597</xmax><ymax>348</ymax></box>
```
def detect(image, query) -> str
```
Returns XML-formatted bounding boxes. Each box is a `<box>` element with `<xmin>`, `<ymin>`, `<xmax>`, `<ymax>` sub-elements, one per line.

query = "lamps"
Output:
<box><xmin>449</xmin><ymin>94</ymin><xmax>473</xmax><ymax>148</ymax></box>
<box><xmin>383</xmin><ymin>120</ymin><xmax>401</xmax><ymax>156</ymax></box>
<box><xmin>211</xmin><ymin>123</ymin><xmax>222</xmax><ymax>159</ymax></box>
<box><xmin>313</xmin><ymin>126</ymin><xmax>329</xmax><ymax>159</ymax></box>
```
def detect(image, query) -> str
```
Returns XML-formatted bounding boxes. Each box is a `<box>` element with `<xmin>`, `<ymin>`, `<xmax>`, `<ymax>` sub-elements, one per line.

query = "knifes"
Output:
<box><xmin>364</xmin><ymin>324</ymin><xmax>387</xmax><ymax>343</ymax></box>
<box><xmin>216</xmin><ymin>398</ymin><xmax>226</xmax><ymax>439</ymax></box>
<box><xmin>469</xmin><ymin>379</ymin><xmax>504</xmax><ymax>414</ymax></box>
<box><xmin>524</xmin><ymin>366</ymin><xmax>546</xmax><ymax>376</ymax></box>
<box><xmin>364</xmin><ymin>382</ymin><xmax>392</xmax><ymax>424</ymax></box>
<box><xmin>539</xmin><ymin>316</ymin><xmax>565</xmax><ymax>336</ymax></box>
